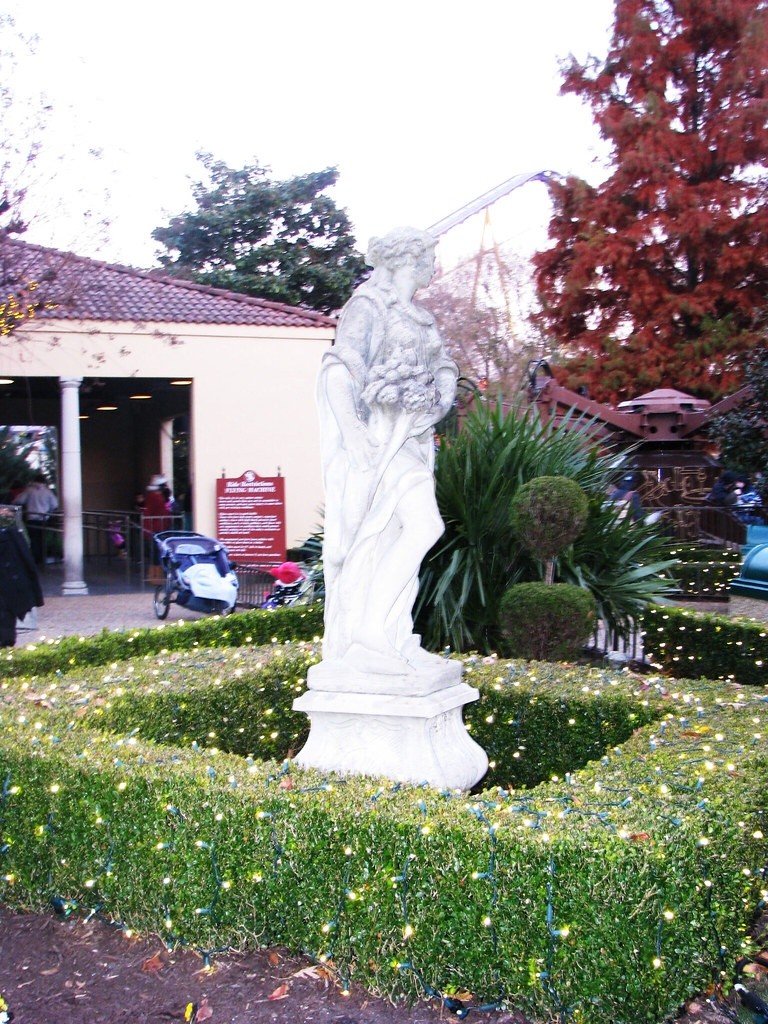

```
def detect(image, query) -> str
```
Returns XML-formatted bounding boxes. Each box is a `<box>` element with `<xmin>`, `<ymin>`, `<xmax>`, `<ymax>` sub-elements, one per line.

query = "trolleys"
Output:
<box><xmin>153</xmin><ymin>530</ymin><xmax>240</xmax><ymax>620</ymax></box>
<box><xmin>261</xmin><ymin>566</ymin><xmax>317</xmax><ymax>613</ymax></box>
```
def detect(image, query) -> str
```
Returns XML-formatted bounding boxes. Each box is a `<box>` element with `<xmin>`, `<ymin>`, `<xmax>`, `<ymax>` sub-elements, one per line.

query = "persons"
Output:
<box><xmin>109</xmin><ymin>474</ymin><xmax>183</xmax><ymax>585</ymax></box>
<box><xmin>7</xmin><ymin>473</ymin><xmax>65</xmax><ymax>566</ymax></box>
<box><xmin>318</xmin><ymin>225</ymin><xmax>455</xmax><ymax>675</ymax></box>
<box><xmin>599</xmin><ymin>471</ymin><xmax>766</xmax><ymax>527</ymax></box>
